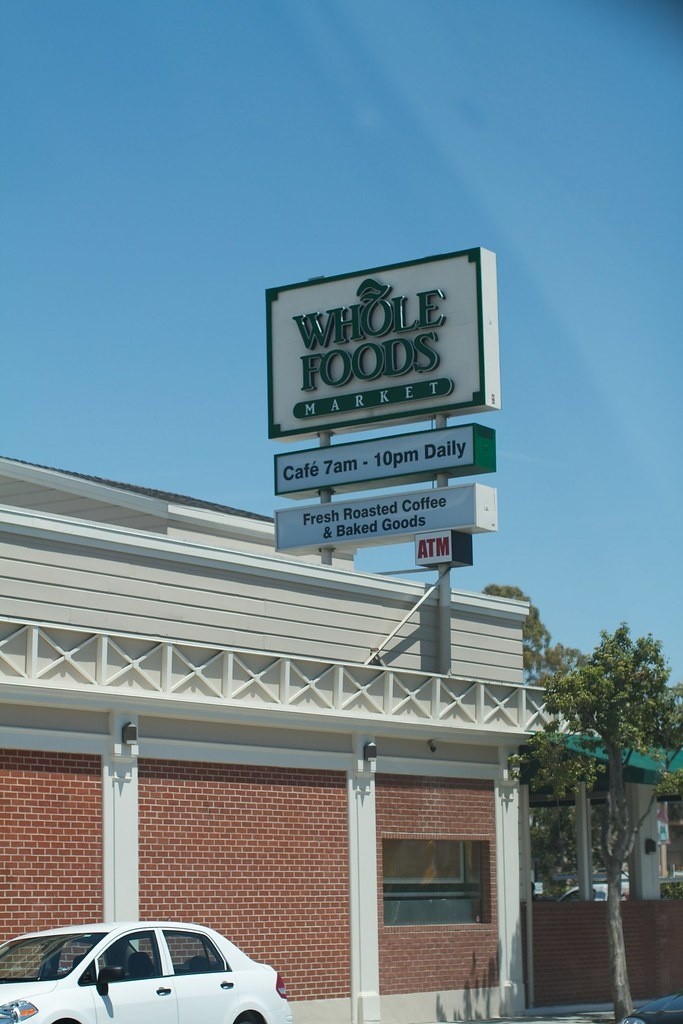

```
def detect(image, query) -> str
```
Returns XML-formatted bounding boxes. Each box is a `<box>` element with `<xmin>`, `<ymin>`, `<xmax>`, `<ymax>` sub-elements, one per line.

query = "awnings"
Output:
<box><xmin>519</xmin><ymin>730</ymin><xmax>683</xmax><ymax>794</ymax></box>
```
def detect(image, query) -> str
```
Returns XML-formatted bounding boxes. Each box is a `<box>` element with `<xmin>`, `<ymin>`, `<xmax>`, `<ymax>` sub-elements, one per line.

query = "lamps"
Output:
<box><xmin>122</xmin><ymin>719</ymin><xmax>137</xmax><ymax>745</ymax></box>
<box><xmin>364</xmin><ymin>743</ymin><xmax>377</xmax><ymax>762</ymax></box>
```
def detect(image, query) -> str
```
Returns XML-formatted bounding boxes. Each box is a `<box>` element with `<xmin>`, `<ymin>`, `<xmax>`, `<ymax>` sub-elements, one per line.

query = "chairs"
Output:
<box><xmin>73</xmin><ymin>952</ymin><xmax>209</xmax><ymax>985</ymax></box>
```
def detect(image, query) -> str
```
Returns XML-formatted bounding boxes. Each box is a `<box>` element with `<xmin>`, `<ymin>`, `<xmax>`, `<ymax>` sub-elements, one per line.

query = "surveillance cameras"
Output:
<box><xmin>429</xmin><ymin>741</ymin><xmax>436</xmax><ymax>752</ymax></box>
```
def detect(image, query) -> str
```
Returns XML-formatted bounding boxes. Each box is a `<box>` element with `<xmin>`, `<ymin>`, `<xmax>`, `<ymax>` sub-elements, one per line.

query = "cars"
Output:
<box><xmin>555</xmin><ymin>882</ymin><xmax>629</xmax><ymax>903</ymax></box>
<box><xmin>618</xmin><ymin>992</ymin><xmax>683</xmax><ymax>1024</ymax></box>
<box><xmin>0</xmin><ymin>921</ymin><xmax>294</xmax><ymax>1024</ymax></box>
